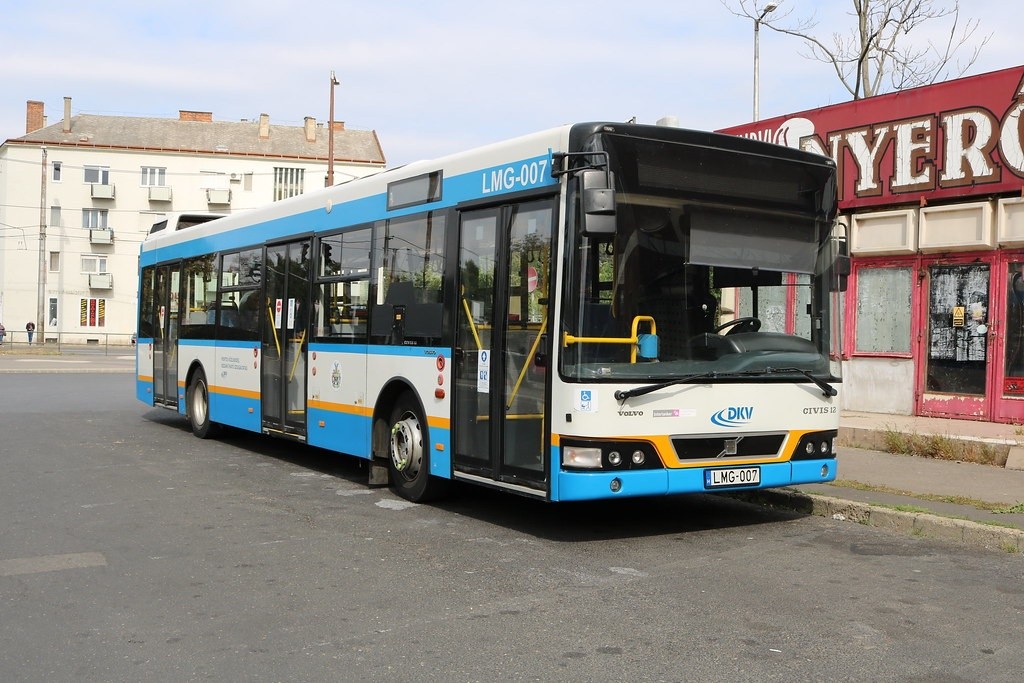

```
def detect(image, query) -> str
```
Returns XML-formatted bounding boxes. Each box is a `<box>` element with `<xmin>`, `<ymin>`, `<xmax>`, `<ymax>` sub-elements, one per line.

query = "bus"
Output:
<box><xmin>133</xmin><ymin>118</ymin><xmax>852</xmax><ymax>506</ymax></box>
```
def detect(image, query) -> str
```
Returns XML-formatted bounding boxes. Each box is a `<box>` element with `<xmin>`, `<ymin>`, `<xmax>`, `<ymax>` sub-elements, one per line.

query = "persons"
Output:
<box><xmin>0</xmin><ymin>323</ymin><xmax>6</xmax><ymax>345</ymax></box>
<box><xmin>26</xmin><ymin>320</ymin><xmax>35</xmax><ymax>346</ymax></box>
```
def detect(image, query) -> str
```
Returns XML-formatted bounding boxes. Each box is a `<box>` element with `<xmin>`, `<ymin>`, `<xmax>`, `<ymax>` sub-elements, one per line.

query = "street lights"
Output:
<box><xmin>327</xmin><ymin>71</ymin><xmax>340</xmax><ymax>186</ymax></box>
<box><xmin>754</xmin><ymin>3</ymin><xmax>778</xmax><ymax>121</ymax></box>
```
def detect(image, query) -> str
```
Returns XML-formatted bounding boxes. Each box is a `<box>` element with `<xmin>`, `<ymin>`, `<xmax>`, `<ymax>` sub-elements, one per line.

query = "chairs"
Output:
<box><xmin>207</xmin><ymin>301</ymin><xmax>241</xmax><ymax>330</ymax></box>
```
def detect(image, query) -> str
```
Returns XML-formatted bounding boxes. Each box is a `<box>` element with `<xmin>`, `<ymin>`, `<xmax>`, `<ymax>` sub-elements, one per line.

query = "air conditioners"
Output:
<box><xmin>229</xmin><ymin>171</ymin><xmax>242</xmax><ymax>181</ymax></box>
